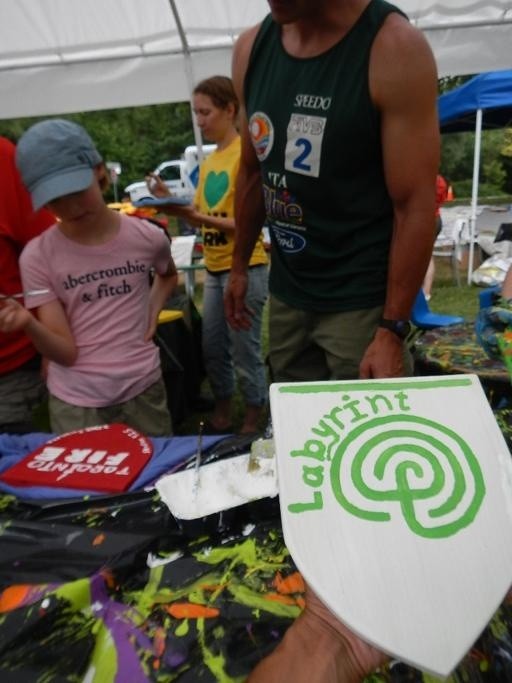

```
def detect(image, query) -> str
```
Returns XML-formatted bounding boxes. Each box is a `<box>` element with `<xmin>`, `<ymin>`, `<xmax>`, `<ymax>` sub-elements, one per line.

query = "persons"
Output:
<box><xmin>224</xmin><ymin>0</ymin><xmax>440</xmax><ymax>382</ymax></box>
<box><xmin>423</xmin><ymin>174</ymin><xmax>447</xmax><ymax>301</ymax></box>
<box><xmin>0</xmin><ymin>126</ymin><xmax>58</xmax><ymax>435</ymax></box>
<box><xmin>142</xmin><ymin>217</ymin><xmax>203</xmax><ymax>341</ymax></box>
<box><xmin>146</xmin><ymin>75</ymin><xmax>288</xmax><ymax>440</ymax></box>
<box><xmin>0</xmin><ymin>120</ymin><xmax>179</xmax><ymax>440</ymax></box>
<box><xmin>244</xmin><ymin>576</ymin><xmax>393</xmax><ymax>683</ymax></box>
<box><xmin>500</xmin><ymin>257</ymin><xmax>512</xmax><ymax>304</ymax></box>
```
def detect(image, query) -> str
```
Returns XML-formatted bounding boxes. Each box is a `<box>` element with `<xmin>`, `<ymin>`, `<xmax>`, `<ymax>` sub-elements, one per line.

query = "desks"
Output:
<box><xmin>410</xmin><ymin>323</ymin><xmax>512</xmax><ymax>408</ymax></box>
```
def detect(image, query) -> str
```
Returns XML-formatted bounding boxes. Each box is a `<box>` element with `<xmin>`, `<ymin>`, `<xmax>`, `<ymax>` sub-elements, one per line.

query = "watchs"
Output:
<box><xmin>377</xmin><ymin>319</ymin><xmax>411</xmax><ymax>337</ymax></box>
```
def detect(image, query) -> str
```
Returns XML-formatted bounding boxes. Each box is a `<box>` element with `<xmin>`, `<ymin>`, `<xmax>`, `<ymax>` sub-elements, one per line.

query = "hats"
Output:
<box><xmin>17</xmin><ymin>117</ymin><xmax>102</xmax><ymax>213</ymax></box>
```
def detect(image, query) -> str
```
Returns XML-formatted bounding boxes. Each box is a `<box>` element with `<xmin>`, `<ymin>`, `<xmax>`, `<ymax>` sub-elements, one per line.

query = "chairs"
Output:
<box><xmin>432</xmin><ymin>220</ymin><xmax>465</xmax><ymax>290</ymax></box>
<box><xmin>155</xmin><ymin>309</ymin><xmax>185</xmax><ymax>369</ymax></box>
<box><xmin>406</xmin><ymin>288</ymin><xmax>463</xmax><ymax>345</ymax></box>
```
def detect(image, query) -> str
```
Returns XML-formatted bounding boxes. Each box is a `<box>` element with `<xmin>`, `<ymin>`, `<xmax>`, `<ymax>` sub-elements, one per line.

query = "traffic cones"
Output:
<box><xmin>445</xmin><ymin>185</ymin><xmax>455</xmax><ymax>202</ymax></box>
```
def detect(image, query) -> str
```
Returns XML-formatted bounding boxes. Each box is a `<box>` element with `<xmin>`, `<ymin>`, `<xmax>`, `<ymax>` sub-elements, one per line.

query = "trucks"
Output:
<box><xmin>124</xmin><ymin>144</ymin><xmax>216</xmax><ymax>203</ymax></box>
